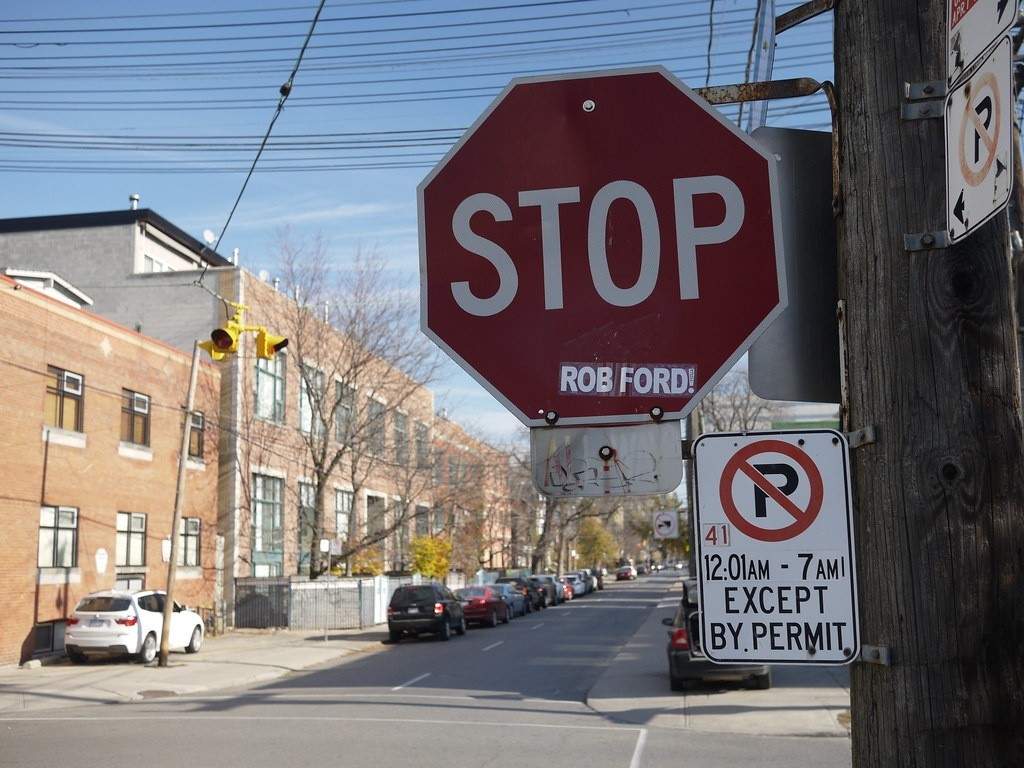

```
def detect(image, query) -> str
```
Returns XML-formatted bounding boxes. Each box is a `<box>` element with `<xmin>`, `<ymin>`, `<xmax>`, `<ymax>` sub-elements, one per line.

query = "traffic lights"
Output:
<box><xmin>263</xmin><ymin>332</ymin><xmax>289</xmax><ymax>360</ymax></box>
<box><xmin>212</xmin><ymin>328</ymin><xmax>238</xmax><ymax>355</ymax></box>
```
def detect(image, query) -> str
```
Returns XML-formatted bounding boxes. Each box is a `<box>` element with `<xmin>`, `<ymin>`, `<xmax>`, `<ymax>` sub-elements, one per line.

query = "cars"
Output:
<box><xmin>489</xmin><ymin>584</ymin><xmax>528</xmax><ymax>618</ymax></box>
<box><xmin>452</xmin><ymin>586</ymin><xmax>510</xmax><ymax>627</ymax></box>
<box><xmin>493</xmin><ymin>567</ymin><xmax>606</xmax><ymax>617</ymax></box>
<box><xmin>617</xmin><ymin>566</ymin><xmax>638</xmax><ymax>581</ymax></box>
<box><xmin>660</xmin><ymin>580</ymin><xmax>774</xmax><ymax>691</ymax></box>
<box><xmin>636</xmin><ymin>563</ymin><xmax>668</xmax><ymax>575</ymax></box>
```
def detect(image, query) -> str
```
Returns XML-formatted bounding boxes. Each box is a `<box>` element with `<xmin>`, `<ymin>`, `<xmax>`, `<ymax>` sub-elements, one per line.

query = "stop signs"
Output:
<box><xmin>411</xmin><ymin>64</ymin><xmax>792</xmax><ymax>429</ymax></box>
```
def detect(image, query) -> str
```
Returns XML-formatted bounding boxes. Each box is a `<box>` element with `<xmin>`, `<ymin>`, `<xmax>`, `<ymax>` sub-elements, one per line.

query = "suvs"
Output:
<box><xmin>64</xmin><ymin>587</ymin><xmax>205</xmax><ymax>664</ymax></box>
<box><xmin>385</xmin><ymin>581</ymin><xmax>466</xmax><ymax>642</ymax></box>
